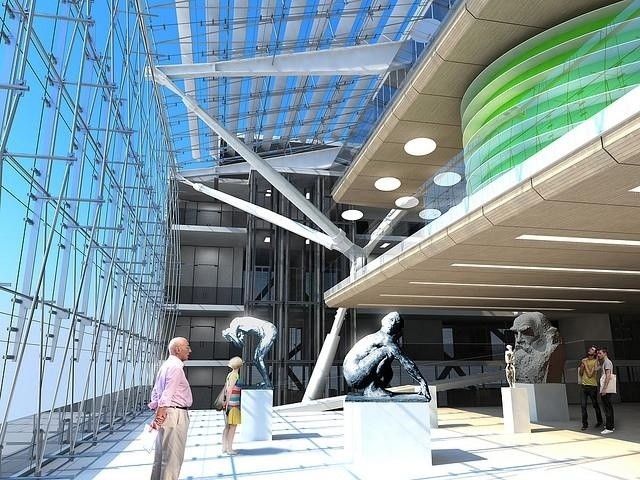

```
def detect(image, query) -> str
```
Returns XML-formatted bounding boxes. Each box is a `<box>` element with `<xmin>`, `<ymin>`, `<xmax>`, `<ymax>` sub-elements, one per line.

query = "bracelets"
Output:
<box><xmin>155</xmin><ymin>415</ymin><xmax>166</xmax><ymax>426</ymax></box>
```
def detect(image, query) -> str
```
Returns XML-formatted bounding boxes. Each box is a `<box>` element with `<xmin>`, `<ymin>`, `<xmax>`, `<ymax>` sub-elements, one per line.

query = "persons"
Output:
<box><xmin>510</xmin><ymin>311</ymin><xmax>564</xmax><ymax>384</ymax></box>
<box><xmin>219</xmin><ymin>315</ymin><xmax>279</xmax><ymax>390</ymax></box>
<box><xmin>343</xmin><ymin>310</ymin><xmax>432</xmax><ymax>400</ymax></box>
<box><xmin>147</xmin><ymin>336</ymin><xmax>194</xmax><ymax>479</ymax></box>
<box><xmin>503</xmin><ymin>344</ymin><xmax>517</xmax><ymax>388</ymax></box>
<box><xmin>213</xmin><ymin>356</ymin><xmax>245</xmax><ymax>456</ymax></box>
<box><xmin>595</xmin><ymin>346</ymin><xmax>616</xmax><ymax>434</ymax></box>
<box><xmin>578</xmin><ymin>343</ymin><xmax>603</xmax><ymax>430</ymax></box>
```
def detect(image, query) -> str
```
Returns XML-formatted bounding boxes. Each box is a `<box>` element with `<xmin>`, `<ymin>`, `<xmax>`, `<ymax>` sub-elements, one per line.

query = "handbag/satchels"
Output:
<box><xmin>578</xmin><ymin>367</ymin><xmax>581</xmax><ymax>384</ymax></box>
<box><xmin>214</xmin><ymin>386</ymin><xmax>224</xmax><ymax>410</ymax></box>
<box><xmin>600</xmin><ymin>374</ymin><xmax>616</xmax><ymax>394</ymax></box>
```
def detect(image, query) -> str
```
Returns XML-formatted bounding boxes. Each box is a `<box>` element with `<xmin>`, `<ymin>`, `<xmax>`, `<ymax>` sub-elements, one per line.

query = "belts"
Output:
<box><xmin>168</xmin><ymin>406</ymin><xmax>187</xmax><ymax>409</ymax></box>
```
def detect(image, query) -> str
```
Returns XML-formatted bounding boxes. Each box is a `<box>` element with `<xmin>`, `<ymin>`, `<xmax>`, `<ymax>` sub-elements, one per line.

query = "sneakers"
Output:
<box><xmin>601</xmin><ymin>428</ymin><xmax>615</xmax><ymax>434</ymax></box>
